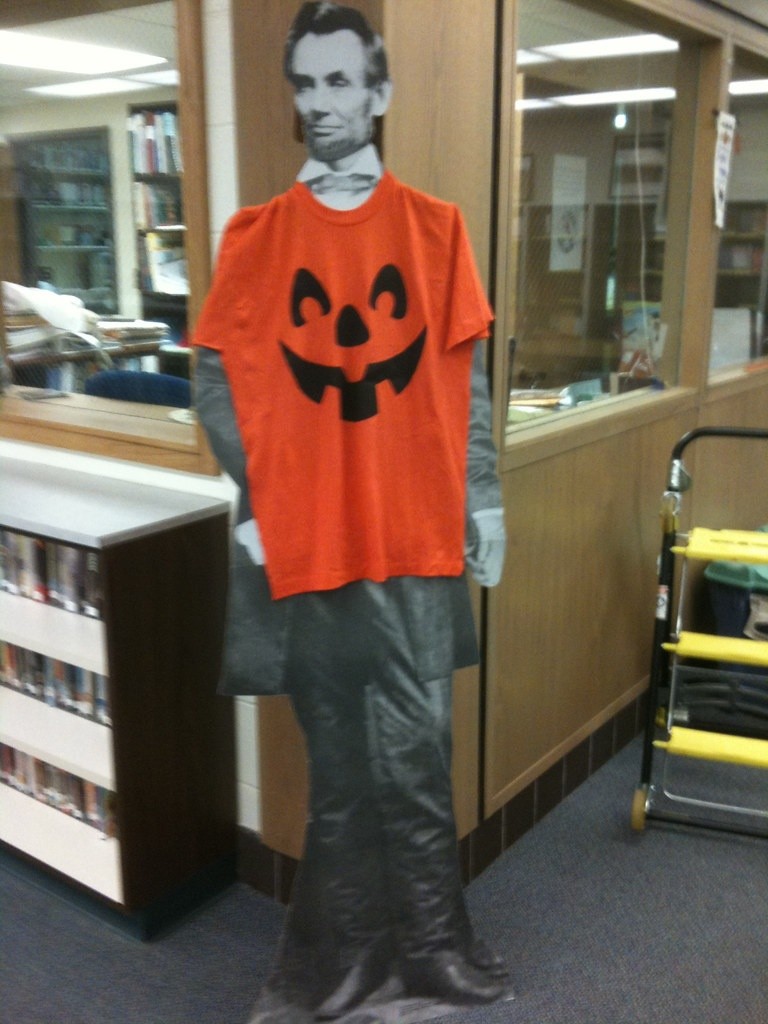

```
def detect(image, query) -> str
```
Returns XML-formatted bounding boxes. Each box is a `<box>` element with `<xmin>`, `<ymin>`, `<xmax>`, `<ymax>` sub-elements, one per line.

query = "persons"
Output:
<box><xmin>189</xmin><ymin>0</ymin><xmax>504</xmax><ymax>1017</ymax></box>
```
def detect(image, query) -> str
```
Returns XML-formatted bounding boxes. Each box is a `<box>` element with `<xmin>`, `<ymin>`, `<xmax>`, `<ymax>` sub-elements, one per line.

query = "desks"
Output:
<box><xmin>51</xmin><ymin>337</ymin><xmax>171</xmax><ymax>388</ymax></box>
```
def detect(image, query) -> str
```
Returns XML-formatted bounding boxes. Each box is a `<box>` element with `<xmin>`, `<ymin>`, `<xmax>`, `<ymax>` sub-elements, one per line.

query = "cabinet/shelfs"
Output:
<box><xmin>0</xmin><ymin>455</ymin><xmax>234</xmax><ymax>948</ymax></box>
<box><xmin>512</xmin><ymin>203</ymin><xmax>594</xmax><ymax>386</ymax></box>
<box><xmin>126</xmin><ymin>98</ymin><xmax>190</xmax><ymax>338</ymax></box>
<box><xmin>640</xmin><ymin>528</ymin><xmax>768</xmax><ymax>824</ymax></box>
<box><xmin>647</xmin><ymin>200</ymin><xmax>768</xmax><ymax>303</ymax></box>
<box><xmin>17</xmin><ymin>130</ymin><xmax>114</xmax><ymax>315</ymax></box>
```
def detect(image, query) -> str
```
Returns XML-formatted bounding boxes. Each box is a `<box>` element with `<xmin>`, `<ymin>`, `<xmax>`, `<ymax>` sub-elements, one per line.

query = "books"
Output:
<box><xmin>0</xmin><ymin>638</ymin><xmax>114</xmax><ymax>728</ymax></box>
<box><xmin>0</xmin><ymin>527</ymin><xmax>106</xmax><ymax>621</ymax></box>
<box><xmin>131</xmin><ymin>108</ymin><xmax>190</xmax><ymax>295</ymax></box>
<box><xmin>717</xmin><ymin>203</ymin><xmax>766</xmax><ymax>271</ymax></box>
<box><xmin>0</xmin><ymin>743</ymin><xmax>120</xmax><ymax>836</ymax></box>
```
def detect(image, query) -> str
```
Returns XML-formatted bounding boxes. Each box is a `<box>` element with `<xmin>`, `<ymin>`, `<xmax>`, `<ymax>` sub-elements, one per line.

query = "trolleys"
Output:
<box><xmin>631</xmin><ymin>425</ymin><xmax>768</xmax><ymax>841</ymax></box>
